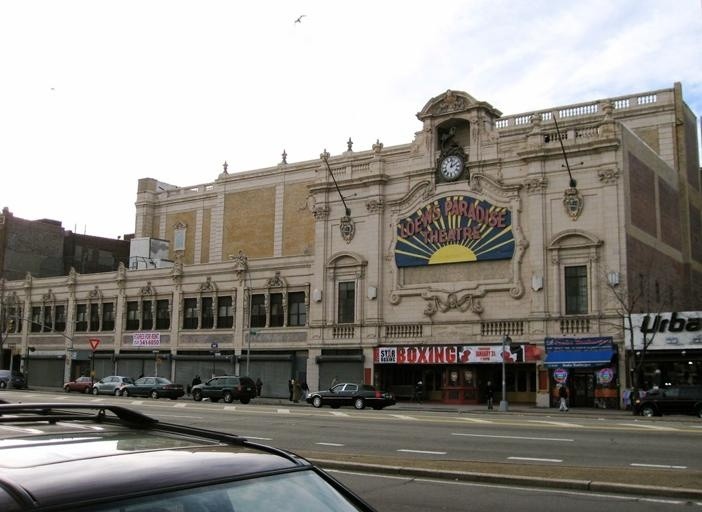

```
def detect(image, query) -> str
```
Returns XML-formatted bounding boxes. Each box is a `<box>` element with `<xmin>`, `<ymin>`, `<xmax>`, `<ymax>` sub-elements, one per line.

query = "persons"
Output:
<box><xmin>256</xmin><ymin>376</ymin><xmax>263</xmax><ymax>397</ymax></box>
<box><xmin>288</xmin><ymin>376</ymin><xmax>310</xmax><ymax>402</ymax></box>
<box><xmin>187</xmin><ymin>374</ymin><xmax>202</xmax><ymax>396</ymax></box>
<box><xmin>558</xmin><ymin>383</ymin><xmax>569</xmax><ymax>411</ymax></box>
<box><xmin>486</xmin><ymin>380</ymin><xmax>494</xmax><ymax>409</ymax></box>
<box><xmin>629</xmin><ymin>386</ymin><xmax>640</xmax><ymax>416</ymax></box>
<box><xmin>416</xmin><ymin>379</ymin><xmax>424</xmax><ymax>405</ymax></box>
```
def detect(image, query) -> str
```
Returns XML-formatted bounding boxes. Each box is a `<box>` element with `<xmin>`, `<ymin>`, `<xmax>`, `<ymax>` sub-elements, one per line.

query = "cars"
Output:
<box><xmin>634</xmin><ymin>384</ymin><xmax>702</xmax><ymax>418</ymax></box>
<box><xmin>306</xmin><ymin>382</ymin><xmax>396</xmax><ymax>410</ymax></box>
<box><xmin>191</xmin><ymin>376</ymin><xmax>258</xmax><ymax>404</ymax></box>
<box><xmin>63</xmin><ymin>376</ymin><xmax>185</xmax><ymax>399</ymax></box>
<box><xmin>0</xmin><ymin>404</ymin><xmax>378</xmax><ymax>512</ymax></box>
<box><xmin>0</xmin><ymin>370</ymin><xmax>25</xmax><ymax>390</ymax></box>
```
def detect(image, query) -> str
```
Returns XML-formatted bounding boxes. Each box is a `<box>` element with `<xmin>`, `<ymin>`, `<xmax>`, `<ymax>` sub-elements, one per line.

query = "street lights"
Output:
<box><xmin>500</xmin><ymin>331</ymin><xmax>511</xmax><ymax>409</ymax></box>
<box><xmin>228</xmin><ymin>254</ymin><xmax>253</xmax><ymax>377</ymax></box>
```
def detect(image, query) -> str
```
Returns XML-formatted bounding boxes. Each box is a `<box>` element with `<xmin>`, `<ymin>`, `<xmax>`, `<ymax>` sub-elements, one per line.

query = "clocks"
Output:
<box><xmin>440</xmin><ymin>155</ymin><xmax>463</xmax><ymax>182</ymax></box>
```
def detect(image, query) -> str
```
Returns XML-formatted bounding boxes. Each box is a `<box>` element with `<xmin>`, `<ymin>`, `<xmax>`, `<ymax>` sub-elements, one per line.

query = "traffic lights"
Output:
<box><xmin>9</xmin><ymin>320</ymin><xmax>14</xmax><ymax>330</ymax></box>
